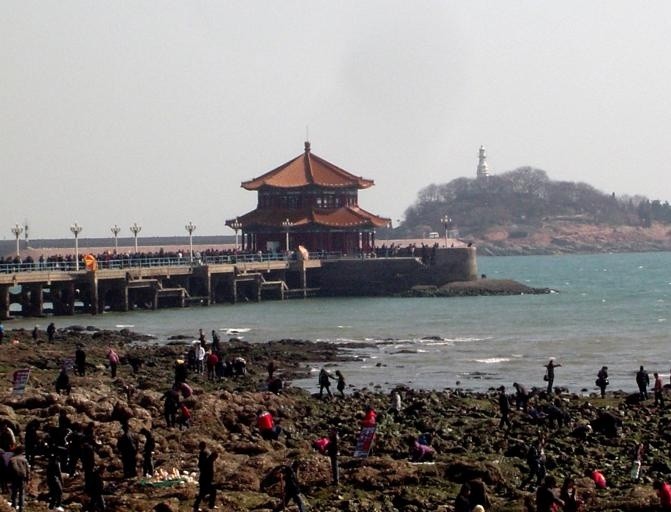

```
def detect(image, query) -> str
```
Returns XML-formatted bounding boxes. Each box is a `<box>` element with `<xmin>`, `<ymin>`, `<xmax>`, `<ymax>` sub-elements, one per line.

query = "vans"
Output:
<box><xmin>429</xmin><ymin>232</ymin><xmax>439</xmax><ymax>238</ymax></box>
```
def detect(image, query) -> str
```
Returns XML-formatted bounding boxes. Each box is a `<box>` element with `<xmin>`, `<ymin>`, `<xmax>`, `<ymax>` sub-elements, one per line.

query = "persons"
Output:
<box><xmin>357</xmin><ymin>405</ymin><xmax>378</xmax><ymax>427</ymax></box>
<box><xmin>632</xmin><ymin>442</ymin><xmax>645</xmax><ymax>484</ymax></box>
<box><xmin>499</xmin><ymin>389</ymin><xmax>516</xmax><ymax>430</ymax></box>
<box><xmin>261</xmin><ymin>361</ymin><xmax>279</xmax><ymax>380</ymax></box>
<box><xmin>637</xmin><ymin>365</ymin><xmax>650</xmax><ymax>400</ymax></box>
<box><xmin>1</xmin><ymin>242</ymin><xmax>487</xmax><ymax>283</ymax></box>
<box><xmin>193</xmin><ymin>448</ymin><xmax>220</xmax><ymax>511</ymax></box>
<box><xmin>109</xmin><ymin>348</ymin><xmax>120</xmax><ymax>377</ymax></box>
<box><xmin>559</xmin><ymin>477</ymin><xmax>579</xmax><ymax>512</ymax></box>
<box><xmin>412</xmin><ymin>440</ymin><xmax>439</xmax><ymax>462</ymax></box>
<box><xmin>653</xmin><ymin>479</ymin><xmax>671</xmax><ymax>511</ymax></box>
<box><xmin>0</xmin><ymin>321</ymin><xmax>5</xmax><ymax>346</ymax></box>
<box><xmin>389</xmin><ymin>389</ymin><xmax>402</xmax><ymax>417</ymax></box>
<box><xmin>46</xmin><ymin>322</ymin><xmax>56</xmax><ymax>343</ymax></box>
<box><xmin>75</xmin><ymin>345</ymin><xmax>86</xmax><ymax>377</ymax></box>
<box><xmin>56</xmin><ymin>367</ymin><xmax>72</xmax><ymax>396</ymax></box>
<box><xmin>455</xmin><ymin>480</ymin><xmax>473</xmax><ymax>511</ymax></box>
<box><xmin>654</xmin><ymin>372</ymin><xmax>665</xmax><ymax>409</ymax></box>
<box><xmin>519</xmin><ymin>439</ymin><xmax>543</xmax><ymax>492</ymax></box>
<box><xmin>584</xmin><ymin>469</ymin><xmax>608</xmax><ymax>489</ymax></box>
<box><xmin>255</xmin><ymin>407</ymin><xmax>282</xmax><ymax>441</ymax></box>
<box><xmin>536</xmin><ymin>431</ymin><xmax>550</xmax><ymax>486</ymax></box>
<box><xmin>271</xmin><ymin>460</ymin><xmax>308</xmax><ymax>512</ymax></box>
<box><xmin>319</xmin><ymin>368</ymin><xmax>336</xmax><ymax>397</ymax></box>
<box><xmin>536</xmin><ymin>475</ymin><xmax>566</xmax><ymax>511</ymax></box>
<box><xmin>312</xmin><ymin>435</ymin><xmax>333</xmax><ymax>454</ymax></box>
<box><xmin>159</xmin><ymin>328</ymin><xmax>247</xmax><ymax>428</ymax></box>
<box><xmin>544</xmin><ymin>359</ymin><xmax>562</xmax><ymax>394</ymax></box>
<box><xmin>335</xmin><ymin>369</ymin><xmax>346</xmax><ymax>397</ymax></box>
<box><xmin>31</xmin><ymin>325</ymin><xmax>38</xmax><ymax>343</ymax></box>
<box><xmin>1</xmin><ymin>409</ymin><xmax>156</xmax><ymax>512</ymax></box>
<box><xmin>513</xmin><ymin>382</ymin><xmax>530</xmax><ymax>412</ymax></box>
<box><xmin>595</xmin><ymin>366</ymin><xmax>610</xmax><ymax>395</ymax></box>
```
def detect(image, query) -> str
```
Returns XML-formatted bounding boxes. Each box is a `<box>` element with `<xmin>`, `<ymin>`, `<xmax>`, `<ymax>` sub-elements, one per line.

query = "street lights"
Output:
<box><xmin>70</xmin><ymin>221</ymin><xmax>83</xmax><ymax>270</ymax></box>
<box><xmin>111</xmin><ymin>225</ymin><xmax>121</xmax><ymax>251</ymax></box>
<box><xmin>185</xmin><ymin>221</ymin><xmax>196</xmax><ymax>261</ymax></box>
<box><xmin>440</xmin><ymin>215</ymin><xmax>452</xmax><ymax>247</ymax></box>
<box><xmin>282</xmin><ymin>219</ymin><xmax>293</xmax><ymax>251</ymax></box>
<box><xmin>129</xmin><ymin>222</ymin><xmax>142</xmax><ymax>253</ymax></box>
<box><xmin>231</xmin><ymin>219</ymin><xmax>242</xmax><ymax>253</ymax></box>
<box><xmin>12</xmin><ymin>223</ymin><xmax>24</xmax><ymax>257</ymax></box>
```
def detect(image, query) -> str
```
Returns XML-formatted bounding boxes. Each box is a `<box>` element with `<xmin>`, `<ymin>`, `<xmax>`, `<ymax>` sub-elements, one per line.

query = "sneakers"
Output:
<box><xmin>1</xmin><ymin>445</ymin><xmax>224</xmax><ymax>511</ymax></box>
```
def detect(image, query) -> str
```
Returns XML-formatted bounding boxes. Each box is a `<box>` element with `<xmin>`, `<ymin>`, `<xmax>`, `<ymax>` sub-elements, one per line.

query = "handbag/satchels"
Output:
<box><xmin>544</xmin><ymin>375</ymin><xmax>550</xmax><ymax>381</ymax></box>
